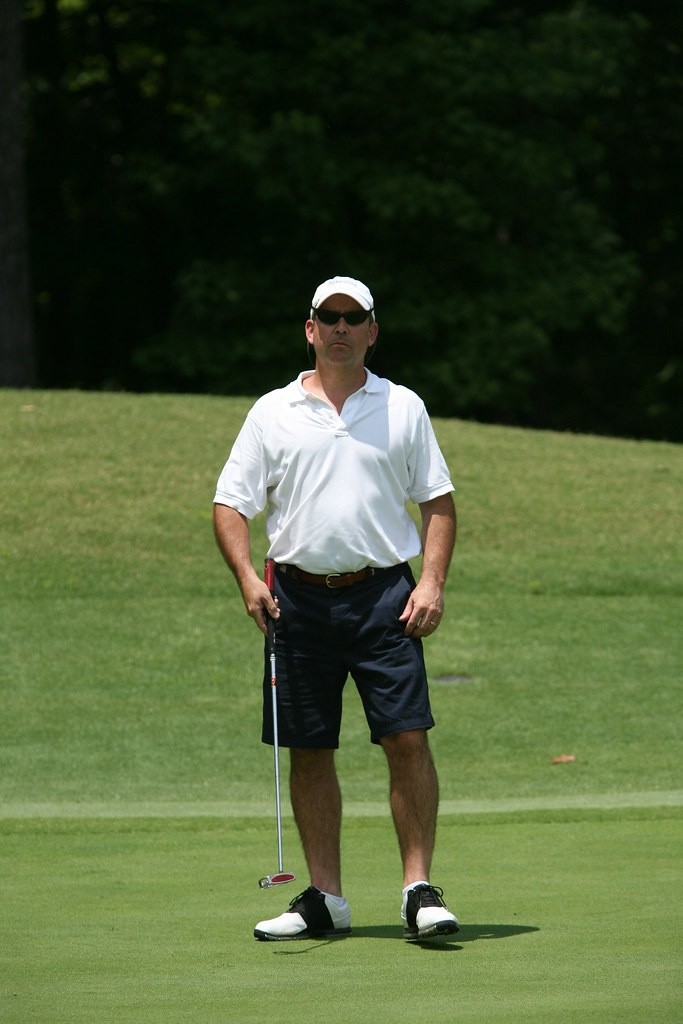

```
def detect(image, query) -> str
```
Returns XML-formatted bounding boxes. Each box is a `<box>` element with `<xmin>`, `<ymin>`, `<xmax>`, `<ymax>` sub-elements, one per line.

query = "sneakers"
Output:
<box><xmin>254</xmin><ymin>886</ymin><xmax>353</xmax><ymax>941</ymax></box>
<box><xmin>400</xmin><ymin>884</ymin><xmax>459</xmax><ymax>939</ymax></box>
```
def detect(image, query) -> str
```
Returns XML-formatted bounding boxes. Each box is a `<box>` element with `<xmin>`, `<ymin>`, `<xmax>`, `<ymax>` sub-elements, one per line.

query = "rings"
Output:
<box><xmin>430</xmin><ymin>621</ymin><xmax>436</xmax><ymax>627</ymax></box>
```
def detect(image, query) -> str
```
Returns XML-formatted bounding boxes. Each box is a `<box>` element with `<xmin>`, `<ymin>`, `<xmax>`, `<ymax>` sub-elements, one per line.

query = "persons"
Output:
<box><xmin>210</xmin><ymin>276</ymin><xmax>462</xmax><ymax>941</ymax></box>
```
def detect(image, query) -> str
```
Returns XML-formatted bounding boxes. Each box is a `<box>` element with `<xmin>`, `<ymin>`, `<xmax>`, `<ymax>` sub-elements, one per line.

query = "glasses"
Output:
<box><xmin>311</xmin><ymin>306</ymin><xmax>374</xmax><ymax>325</ymax></box>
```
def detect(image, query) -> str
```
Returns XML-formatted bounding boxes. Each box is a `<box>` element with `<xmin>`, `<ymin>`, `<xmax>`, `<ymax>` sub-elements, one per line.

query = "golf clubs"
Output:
<box><xmin>255</xmin><ymin>557</ymin><xmax>297</xmax><ymax>888</ymax></box>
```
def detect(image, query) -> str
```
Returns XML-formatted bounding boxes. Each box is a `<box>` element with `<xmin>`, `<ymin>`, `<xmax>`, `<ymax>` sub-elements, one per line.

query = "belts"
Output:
<box><xmin>279</xmin><ymin>564</ymin><xmax>388</xmax><ymax>588</ymax></box>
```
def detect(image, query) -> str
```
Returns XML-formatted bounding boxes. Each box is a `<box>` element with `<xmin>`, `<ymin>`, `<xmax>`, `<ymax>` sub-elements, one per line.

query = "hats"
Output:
<box><xmin>310</xmin><ymin>276</ymin><xmax>375</xmax><ymax>324</ymax></box>
